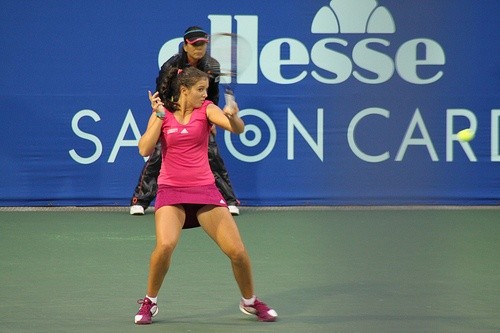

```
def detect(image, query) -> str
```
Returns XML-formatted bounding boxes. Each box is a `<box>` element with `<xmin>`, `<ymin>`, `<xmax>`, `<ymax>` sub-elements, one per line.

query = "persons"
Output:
<box><xmin>129</xmin><ymin>26</ymin><xmax>240</xmax><ymax>216</ymax></box>
<box><xmin>134</xmin><ymin>67</ymin><xmax>278</xmax><ymax>326</ymax></box>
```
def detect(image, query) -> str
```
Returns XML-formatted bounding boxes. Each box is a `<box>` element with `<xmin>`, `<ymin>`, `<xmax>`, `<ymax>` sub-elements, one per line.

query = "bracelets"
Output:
<box><xmin>156</xmin><ymin>112</ymin><xmax>167</xmax><ymax>121</ymax></box>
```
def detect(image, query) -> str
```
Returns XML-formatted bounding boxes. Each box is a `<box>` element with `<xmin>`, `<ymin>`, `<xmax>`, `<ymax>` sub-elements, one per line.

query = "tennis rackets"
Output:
<box><xmin>202</xmin><ymin>31</ymin><xmax>251</xmax><ymax>118</ymax></box>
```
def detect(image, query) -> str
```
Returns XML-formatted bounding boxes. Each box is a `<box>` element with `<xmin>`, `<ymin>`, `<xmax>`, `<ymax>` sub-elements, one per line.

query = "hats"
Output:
<box><xmin>184</xmin><ymin>29</ymin><xmax>209</xmax><ymax>45</ymax></box>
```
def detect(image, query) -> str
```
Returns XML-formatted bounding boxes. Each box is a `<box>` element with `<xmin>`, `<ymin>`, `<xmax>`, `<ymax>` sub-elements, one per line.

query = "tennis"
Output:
<box><xmin>457</xmin><ymin>129</ymin><xmax>476</xmax><ymax>142</ymax></box>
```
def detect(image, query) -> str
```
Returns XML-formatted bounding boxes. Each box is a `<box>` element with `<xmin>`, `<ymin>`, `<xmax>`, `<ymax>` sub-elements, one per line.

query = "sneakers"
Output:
<box><xmin>228</xmin><ymin>205</ymin><xmax>240</xmax><ymax>216</ymax></box>
<box><xmin>239</xmin><ymin>297</ymin><xmax>278</xmax><ymax>322</ymax></box>
<box><xmin>134</xmin><ymin>297</ymin><xmax>158</xmax><ymax>323</ymax></box>
<box><xmin>130</xmin><ymin>205</ymin><xmax>144</xmax><ymax>215</ymax></box>
